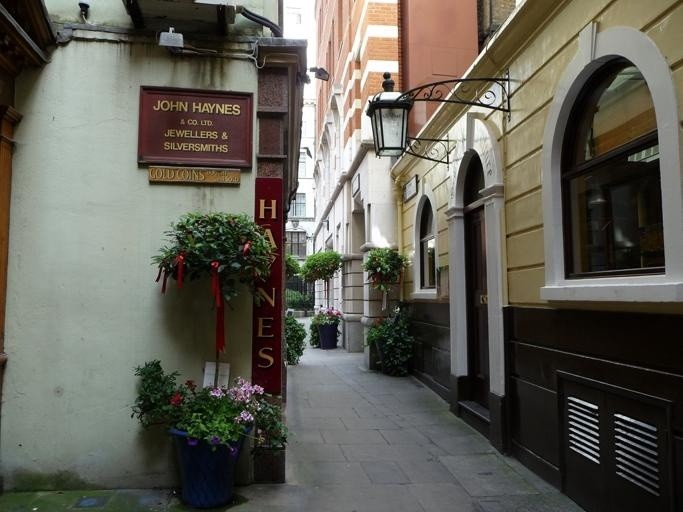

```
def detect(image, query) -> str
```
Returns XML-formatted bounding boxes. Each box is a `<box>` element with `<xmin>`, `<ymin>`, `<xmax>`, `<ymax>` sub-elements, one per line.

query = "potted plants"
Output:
<box><xmin>366</xmin><ymin>304</ymin><xmax>424</xmax><ymax>375</ymax></box>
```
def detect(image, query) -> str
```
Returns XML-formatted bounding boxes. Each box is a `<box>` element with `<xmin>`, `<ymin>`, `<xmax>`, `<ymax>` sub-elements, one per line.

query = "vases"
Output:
<box><xmin>316</xmin><ymin>324</ymin><xmax>337</xmax><ymax>349</ymax></box>
<box><xmin>169</xmin><ymin>424</ymin><xmax>250</xmax><ymax>506</ymax></box>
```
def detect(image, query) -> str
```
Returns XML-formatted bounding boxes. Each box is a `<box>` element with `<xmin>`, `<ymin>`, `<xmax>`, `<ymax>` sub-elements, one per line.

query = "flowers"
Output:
<box><xmin>308</xmin><ymin>309</ymin><xmax>341</xmax><ymax>350</ymax></box>
<box><xmin>132</xmin><ymin>360</ymin><xmax>265</xmax><ymax>456</ymax></box>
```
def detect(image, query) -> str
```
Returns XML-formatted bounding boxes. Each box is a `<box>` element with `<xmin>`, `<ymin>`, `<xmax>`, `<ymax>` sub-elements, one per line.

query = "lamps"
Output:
<box><xmin>365</xmin><ymin>67</ymin><xmax>511</xmax><ymax>165</ymax></box>
